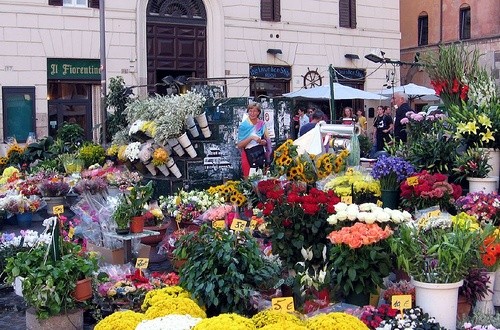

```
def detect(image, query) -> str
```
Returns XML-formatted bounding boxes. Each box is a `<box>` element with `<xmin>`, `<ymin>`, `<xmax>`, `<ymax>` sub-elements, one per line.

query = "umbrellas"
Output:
<box><xmin>282</xmin><ymin>82</ymin><xmax>387</xmax><ymax>100</ymax></box>
<box><xmin>377</xmin><ymin>82</ymin><xmax>438</xmax><ymax>111</ymax></box>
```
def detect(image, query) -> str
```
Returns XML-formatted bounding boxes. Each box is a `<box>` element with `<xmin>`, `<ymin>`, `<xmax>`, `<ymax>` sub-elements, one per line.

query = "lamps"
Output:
<box><xmin>162</xmin><ymin>76</ymin><xmax>174</xmax><ymax>85</ymax></box>
<box><xmin>267</xmin><ymin>49</ymin><xmax>282</xmax><ymax>53</ymax></box>
<box><xmin>345</xmin><ymin>54</ymin><xmax>360</xmax><ymax>59</ymax></box>
<box><xmin>174</xmin><ymin>75</ymin><xmax>188</xmax><ymax>84</ymax></box>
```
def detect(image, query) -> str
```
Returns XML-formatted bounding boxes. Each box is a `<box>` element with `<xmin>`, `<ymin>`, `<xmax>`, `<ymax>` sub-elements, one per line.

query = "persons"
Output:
<box><xmin>373</xmin><ymin>105</ymin><xmax>394</xmax><ymax>152</ymax></box>
<box><xmin>298</xmin><ymin>106</ymin><xmax>331</xmax><ymax>152</ymax></box>
<box><xmin>237</xmin><ymin>102</ymin><xmax>270</xmax><ymax>176</ymax></box>
<box><xmin>391</xmin><ymin>90</ymin><xmax>417</xmax><ymax>144</ymax></box>
<box><xmin>342</xmin><ymin>107</ymin><xmax>367</xmax><ymax>130</ymax></box>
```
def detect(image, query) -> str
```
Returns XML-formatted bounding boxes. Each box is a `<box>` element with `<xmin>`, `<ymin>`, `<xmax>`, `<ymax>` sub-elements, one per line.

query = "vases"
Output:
<box><xmin>492</xmin><ymin>268</ymin><xmax>500</xmax><ymax>306</ymax></box>
<box><xmin>458</xmin><ymin>294</ymin><xmax>471</xmax><ymax>319</ymax></box>
<box><xmin>128</xmin><ymin>217</ymin><xmax>187</xmax><ymax>269</ymax></box>
<box><xmin>475</xmin><ymin>272</ymin><xmax>495</xmax><ymax>316</ymax></box>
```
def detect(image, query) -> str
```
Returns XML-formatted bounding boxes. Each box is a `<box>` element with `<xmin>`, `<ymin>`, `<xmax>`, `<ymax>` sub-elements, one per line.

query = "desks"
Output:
<box><xmin>102</xmin><ymin>229</ymin><xmax>160</xmax><ymax>262</ymax></box>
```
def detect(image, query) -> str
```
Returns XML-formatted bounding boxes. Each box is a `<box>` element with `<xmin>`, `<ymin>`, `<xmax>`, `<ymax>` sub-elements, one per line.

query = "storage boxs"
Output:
<box><xmin>87</xmin><ymin>241</ymin><xmax>161</xmax><ymax>264</ymax></box>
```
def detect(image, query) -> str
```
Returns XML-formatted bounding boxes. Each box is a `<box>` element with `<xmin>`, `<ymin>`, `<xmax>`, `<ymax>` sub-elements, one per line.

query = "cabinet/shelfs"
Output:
<box><xmin>139</xmin><ymin>97</ymin><xmax>248</xmax><ymax>196</ymax></box>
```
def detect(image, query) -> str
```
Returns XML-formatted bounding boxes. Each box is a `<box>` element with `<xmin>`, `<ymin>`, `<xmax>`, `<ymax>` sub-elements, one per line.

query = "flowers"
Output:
<box><xmin>149</xmin><ymin>39</ymin><xmax>500</xmax><ymax>307</ymax></box>
<box><xmin>0</xmin><ymin>38</ymin><xmax>205</xmax><ymax>221</ymax></box>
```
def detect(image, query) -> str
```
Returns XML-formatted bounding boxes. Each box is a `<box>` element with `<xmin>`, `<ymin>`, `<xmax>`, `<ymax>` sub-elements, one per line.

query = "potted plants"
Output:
<box><xmin>112</xmin><ymin>195</ymin><xmax>133</xmax><ymax>235</ymax></box>
<box><xmin>0</xmin><ymin>239</ymin><xmax>109</xmax><ymax>323</ymax></box>
